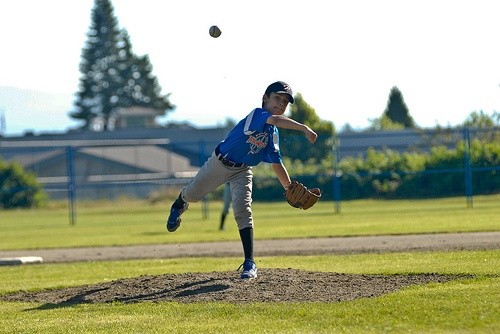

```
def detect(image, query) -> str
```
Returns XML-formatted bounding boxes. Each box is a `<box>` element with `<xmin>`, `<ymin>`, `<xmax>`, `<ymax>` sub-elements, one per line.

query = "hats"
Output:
<box><xmin>267</xmin><ymin>81</ymin><xmax>295</xmax><ymax>105</ymax></box>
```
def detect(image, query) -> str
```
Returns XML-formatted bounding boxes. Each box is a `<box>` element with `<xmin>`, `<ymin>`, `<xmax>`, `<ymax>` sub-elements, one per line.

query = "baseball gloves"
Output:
<box><xmin>286</xmin><ymin>179</ymin><xmax>321</xmax><ymax>211</ymax></box>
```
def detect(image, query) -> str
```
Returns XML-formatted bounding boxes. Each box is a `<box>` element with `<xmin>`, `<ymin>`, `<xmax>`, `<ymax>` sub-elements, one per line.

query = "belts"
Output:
<box><xmin>215</xmin><ymin>146</ymin><xmax>249</xmax><ymax>168</ymax></box>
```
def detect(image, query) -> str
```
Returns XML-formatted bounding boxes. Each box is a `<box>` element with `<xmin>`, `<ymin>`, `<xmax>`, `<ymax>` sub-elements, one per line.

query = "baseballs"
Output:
<box><xmin>209</xmin><ymin>24</ymin><xmax>222</xmax><ymax>39</ymax></box>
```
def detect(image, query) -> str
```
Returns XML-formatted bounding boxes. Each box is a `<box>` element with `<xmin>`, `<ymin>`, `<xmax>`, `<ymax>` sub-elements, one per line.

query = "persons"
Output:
<box><xmin>167</xmin><ymin>80</ymin><xmax>317</xmax><ymax>278</ymax></box>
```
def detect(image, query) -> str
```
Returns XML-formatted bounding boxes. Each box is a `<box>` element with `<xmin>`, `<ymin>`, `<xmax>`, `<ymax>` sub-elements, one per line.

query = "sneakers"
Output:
<box><xmin>236</xmin><ymin>259</ymin><xmax>258</xmax><ymax>280</ymax></box>
<box><xmin>166</xmin><ymin>193</ymin><xmax>189</xmax><ymax>232</ymax></box>
